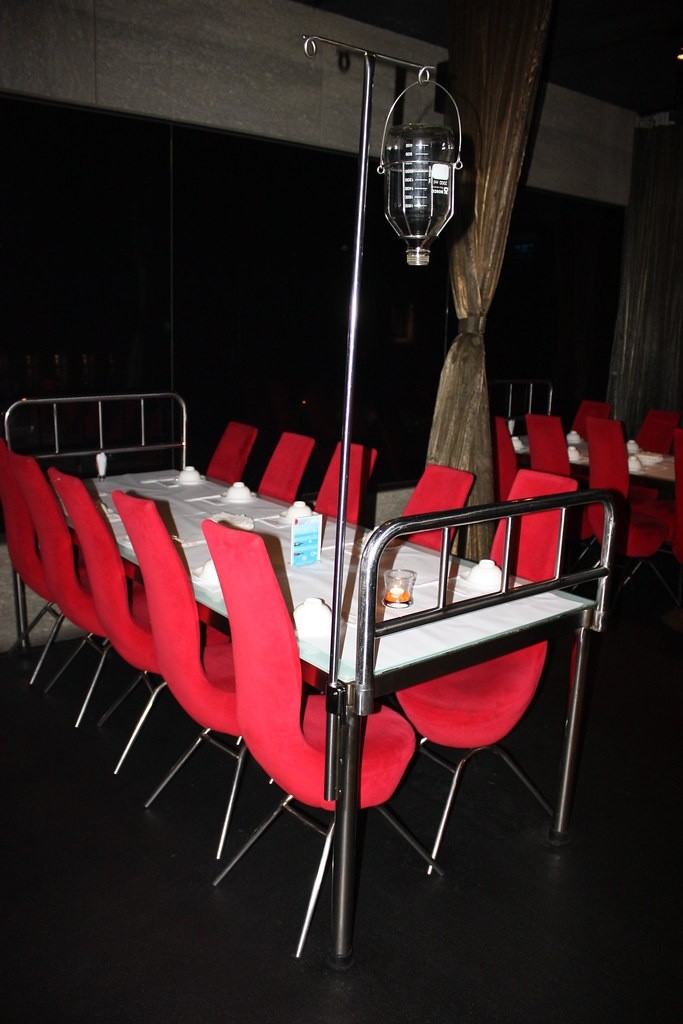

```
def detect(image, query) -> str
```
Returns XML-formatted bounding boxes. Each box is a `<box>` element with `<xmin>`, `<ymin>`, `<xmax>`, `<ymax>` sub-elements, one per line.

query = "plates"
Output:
<box><xmin>175</xmin><ymin>475</ymin><xmax>206</xmax><ymax>485</ymax></box>
<box><xmin>279</xmin><ymin>510</ymin><xmax>318</xmax><ymax>521</ymax></box>
<box><xmin>457</xmin><ymin>567</ymin><xmax>488</xmax><ymax>593</ymax></box>
<box><xmin>191</xmin><ymin>566</ymin><xmax>220</xmax><ymax>594</ymax></box>
<box><xmin>218</xmin><ymin>490</ymin><xmax>256</xmax><ymax>502</ymax></box>
<box><xmin>354</xmin><ymin>537</ymin><xmax>404</xmax><ymax>550</ymax></box>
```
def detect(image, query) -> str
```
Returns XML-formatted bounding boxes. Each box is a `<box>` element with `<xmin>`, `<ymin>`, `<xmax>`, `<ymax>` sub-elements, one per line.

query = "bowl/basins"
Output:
<box><xmin>510</xmin><ymin>430</ymin><xmax>663</xmax><ymax>471</ymax></box>
<box><xmin>200</xmin><ymin>558</ymin><xmax>218</xmax><ymax>584</ymax></box>
<box><xmin>468</xmin><ymin>558</ymin><xmax>502</xmax><ymax>592</ymax></box>
<box><xmin>179</xmin><ymin>467</ymin><xmax>200</xmax><ymax>482</ymax></box>
<box><xmin>227</xmin><ymin>482</ymin><xmax>251</xmax><ymax>500</ymax></box>
<box><xmin>290</xmin><ymin>598</ymin><xmax>332</xmax><ymax>637</ymax></box>
<box><xmin>287</xmin><ymin>501</ymin><xmax>312</xmax><ymax>518</ymax></box>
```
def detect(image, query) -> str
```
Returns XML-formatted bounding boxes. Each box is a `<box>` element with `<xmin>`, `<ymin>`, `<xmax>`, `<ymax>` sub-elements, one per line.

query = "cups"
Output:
<box><xmin>95</xmin><ymin>454</ymin><xmax>107</xmax><ymax>482</ymax></box>
<box><xmin>381</xmin><ymin>570</ymin><xmax>416</xmax><ymax>609</ymax></box>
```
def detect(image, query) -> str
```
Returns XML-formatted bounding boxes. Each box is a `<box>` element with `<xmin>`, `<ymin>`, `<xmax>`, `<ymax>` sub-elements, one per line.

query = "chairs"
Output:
<box><xmin>0</xmin><ymin>401</ymin><xmax>683</xmax><ymax>958</ymax></box>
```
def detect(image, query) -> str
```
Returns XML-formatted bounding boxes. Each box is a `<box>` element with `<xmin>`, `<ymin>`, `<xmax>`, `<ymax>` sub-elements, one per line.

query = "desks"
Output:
<box><xmin>0</xmin><ymin>392</ymin><xmax>632</xmax><ymax>956</ymax></box>
<box><xmin>511</xmin><ymin>430</ymin><xmax>676</xmax><ymax>497</ymax></box>
